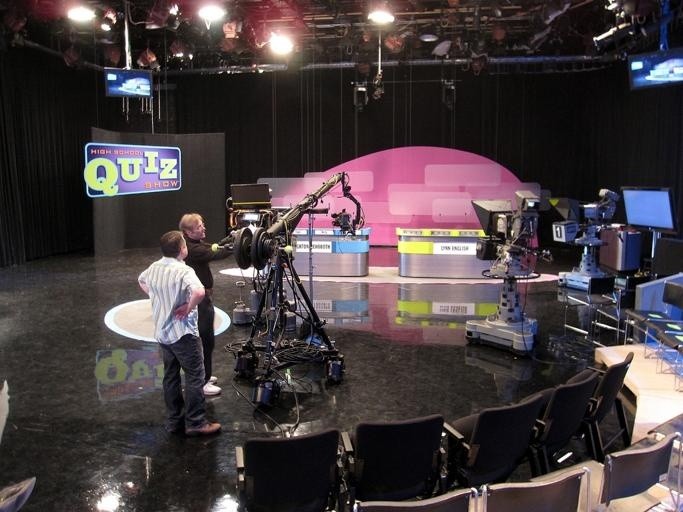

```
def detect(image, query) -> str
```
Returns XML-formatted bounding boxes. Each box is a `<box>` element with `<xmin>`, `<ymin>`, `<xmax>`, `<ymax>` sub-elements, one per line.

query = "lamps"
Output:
<box><xmin>0</xmin><ymin>0</ymin><xmax>651</xmax><ymax>112</ymax></box>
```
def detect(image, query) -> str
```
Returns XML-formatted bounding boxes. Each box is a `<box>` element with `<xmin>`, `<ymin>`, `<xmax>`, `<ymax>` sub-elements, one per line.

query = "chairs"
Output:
<box><xmin>235</xmin><ymin>276</ymin><xmax>683</xmax><ymax>511</ymax></box>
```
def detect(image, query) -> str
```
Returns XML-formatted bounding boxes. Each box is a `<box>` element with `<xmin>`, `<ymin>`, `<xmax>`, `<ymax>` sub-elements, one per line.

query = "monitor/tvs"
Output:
<box><xmin>230</xmin><ymin>184</ymin><xmax>271</xmax><ymax>210</ymax></box>
<box><xmin>628</xmin><ymin>48</ymin><xmax>683</xmax><ymax>92</ymax></box>
<box><xmin>104</xmin><ymin>67</ymin><xmax>154</xmax><ymax>98</ymax></box>
<box><xmin>620</xmin><ymin>187</ymin><xmax>680</xmax><ymax>236</ymax></box>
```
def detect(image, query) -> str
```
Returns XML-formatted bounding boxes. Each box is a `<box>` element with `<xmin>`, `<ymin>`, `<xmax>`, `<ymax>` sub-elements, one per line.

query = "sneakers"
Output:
<box><xmin>208</xmin><ymin>376</ymin><xmax>217</xmax><ymax>385</ymax></box>
<box><xmin>203</xmin><ymin>382</ymin><xmax>222</xmax><ymax>395</ymax></box>
<box><xmin>168</xmin><ymin>420</ymin><xmax>222</xmax><ymax>436</ymax></box>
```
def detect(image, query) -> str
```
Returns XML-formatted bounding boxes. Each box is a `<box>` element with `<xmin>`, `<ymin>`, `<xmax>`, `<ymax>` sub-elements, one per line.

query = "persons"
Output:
<box><xmin>137</xmin><ymin>229</ymin><xmax>222</xmax><ymax>435</ymax></box>
<box><xmin>178</xmin><ymin>212</ymin><xmax>234</xmax><ymax>397</ymax></box>
